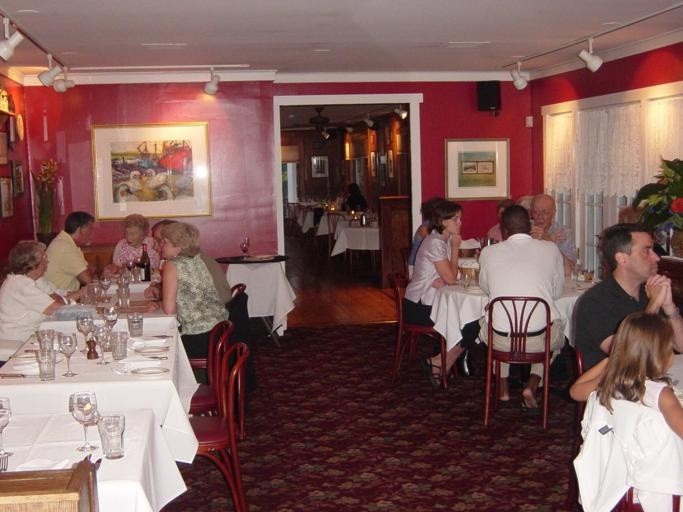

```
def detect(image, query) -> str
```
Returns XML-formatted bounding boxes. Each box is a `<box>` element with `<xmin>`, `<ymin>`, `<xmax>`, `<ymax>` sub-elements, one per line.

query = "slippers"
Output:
<box><xmin>521</xmin><ymin>403</ymin><xmax>541</xmax><ymax>415</ymax></box>
<box><xmin>499</xmin><ymin>400</ymin><xmax>510</xmax><ymax>407</ymax></box>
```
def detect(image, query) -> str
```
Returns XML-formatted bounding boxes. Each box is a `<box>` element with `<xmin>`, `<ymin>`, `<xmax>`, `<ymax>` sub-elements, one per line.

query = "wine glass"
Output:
<box><xmin>239</xmin><ymin>241</ymin><xmax>249</xmax><ymax>258</ymax></box>
<box><xmin>0</xmin><ymin>398</ymin><xmax>14</xmax><ymax>460</ymax></box>
<box><xmin>126</xmin><ymin>257</ymin><xmax>140</xmax><ymax>281</ymax></box>
<box><xmin>69</xmin><ymin>392</ymin><xmax>99</xmax><ymax>453</ymax></box>
<box><xmin>79</xmin><ymin>272</ymin><xmax>115</xmax><ymax>310</ymax></box>
<box><xmin>75</xmin><ymin>304</ymin><xmax>119</xmax><ymax>370</ymax></box>
<box><xmin>54</xmin><ymin>331</ymin><xmax>77</xmax><ymax>381</ymax></box>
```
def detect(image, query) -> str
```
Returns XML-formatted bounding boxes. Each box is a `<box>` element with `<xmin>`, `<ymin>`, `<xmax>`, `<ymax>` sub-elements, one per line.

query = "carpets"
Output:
<box><xmin>159</xmin><ymin>319</ymin><xmax>584</xmax><ymax>511</ymax></box>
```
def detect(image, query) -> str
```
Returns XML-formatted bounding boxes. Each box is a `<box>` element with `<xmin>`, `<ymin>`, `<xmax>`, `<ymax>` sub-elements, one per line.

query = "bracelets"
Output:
<box><xmin>60</xmin><ymin>295</ymin><xmax>67</xmax><ymax>306</ymax></box>
<box><xmin>664</xmin><ymin>306</ymin><xmax>680</xmax><ymax>319</ymax></box>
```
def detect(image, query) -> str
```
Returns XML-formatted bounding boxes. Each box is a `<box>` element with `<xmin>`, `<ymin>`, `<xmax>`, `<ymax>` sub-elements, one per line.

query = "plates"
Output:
<box><xmin>133</xmin><ymin>346</ymin><xmax>170</xmax><ymax>354</ymax></box>
<box><xmin>130</xmin><ymin>367</ymin><xmax>169</xmax><ymax>378</ymax></box>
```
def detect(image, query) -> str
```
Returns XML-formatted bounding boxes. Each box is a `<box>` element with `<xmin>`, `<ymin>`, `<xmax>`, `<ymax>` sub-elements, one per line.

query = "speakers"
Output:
<box><xmin>326</xmin><ymin>128</ymin><xmax>336</xmax><ymax>141</ymax></box>
<box><xmin>477</xmin><ymin>81</ymin><xmax>502</xmax><ymax>110</ymax></box>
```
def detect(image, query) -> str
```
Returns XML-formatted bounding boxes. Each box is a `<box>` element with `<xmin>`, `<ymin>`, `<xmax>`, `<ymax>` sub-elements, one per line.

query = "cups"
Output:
<box><xmin>126</xmin><ymin>311</ymin><xmax>143</xmax><ymax>337</ymax></box>
<box><xmin>108</xmin><ymin>331</ymin><xmax>128</xmax><ymax>361</ymax></box>
<box><xmin>116</xmin><ymin>277</ymin><xmax>130</xmax><ymax>310</ymax></box>
<box><xmin>461</xmin><ymin>270</ymin><xmax>474</xmax><ymax>287</ymax></box>
<box><xmin>95</xmin><ymin>414</ymin><xmax>126</xmax><ymax>460</ymax></box>
<box><xmin>34</xmin><ymin>328</ymin><xmax>57</xmax><ymax>384</ymax></box>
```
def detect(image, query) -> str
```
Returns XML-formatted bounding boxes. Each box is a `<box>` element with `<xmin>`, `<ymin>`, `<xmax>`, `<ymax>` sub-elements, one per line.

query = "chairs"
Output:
<box><xmin>186</xmin><ymin>339</ymin><xmax>251</xmax><ymax>510</ymax></box>
<box><xmin>186</xmin><ymin>319</ymin><xmax>237</xmax><ymax>472</ymax></box>
<box><xmin>389</xmin><ymin>246</ymin><xmax>683</xmax><ymax>511</ymax></box>
<box><xmin>0</xmin><ymin>246</ymin><xmax>289</xmax><ymax>512</ymax></box>
<box><xmin>284</xmin><ymin>199</ymin><xmax>381</xmax><ymax>274</ymax></box>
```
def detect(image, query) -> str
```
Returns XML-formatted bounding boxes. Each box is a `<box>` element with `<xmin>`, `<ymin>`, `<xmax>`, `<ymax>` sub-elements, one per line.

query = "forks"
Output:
<box><xmin>0</xmin><ymin>455</ymin><xmax>8</xmax><ymax>471</ymax></box>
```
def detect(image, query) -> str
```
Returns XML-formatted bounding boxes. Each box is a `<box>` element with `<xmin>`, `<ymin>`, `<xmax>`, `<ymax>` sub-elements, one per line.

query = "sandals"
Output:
<box><xmin>424</xmin><ymin>356</ymin><xmax>442</xmax><ymax>390</ymax></box>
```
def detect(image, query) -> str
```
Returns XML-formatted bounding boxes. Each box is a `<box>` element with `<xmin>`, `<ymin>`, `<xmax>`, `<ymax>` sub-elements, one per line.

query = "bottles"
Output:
<box><xmin>139</xmin><ymin>243</ymin><xmax>151</xmax><ymax>282</ymax></box>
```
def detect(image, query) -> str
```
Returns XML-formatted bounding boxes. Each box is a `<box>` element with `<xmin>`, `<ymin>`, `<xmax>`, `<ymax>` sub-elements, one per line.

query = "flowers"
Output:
<box><xmin>631</xmin><ymin>155</ymin><xmax>683</xmax><ymax>256</ymax></box>
<box><xmin>31</xmin><ymin>157</ymin><xmax>58</xmax><ymax>234</ymax></box>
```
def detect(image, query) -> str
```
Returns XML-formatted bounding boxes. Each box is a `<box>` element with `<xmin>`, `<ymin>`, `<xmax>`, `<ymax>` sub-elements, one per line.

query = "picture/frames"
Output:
<box><xmin>13</xmin><ymin>159</ymin><xmax>24</xmax><ymax>197</ymax></box>
<box><xmin>90</xmin><ymin>121</ymin><xmax>212</xmax><ymax>222</ymax></box>
<box><xmin>0</xmin><ymin>132</ymin><xmax>8</xmax><ymax>165</ymax></box>
<box><xmin>311</xmin><ymin>156</ymin><xmax>329</xmax><ymax>179</ymax></box>
<box><xmin>387</xmin><ymin>150</ymin><xmax>394</xmax><ymax>179</ymax></box>
<box><xmin>371</xmin><ymin>152</ymin><xmax>376</xmax><ymax>178</ymax></box>
<box><xmin>444</xmin><ymin>138</ymin><xmax>510</xmax><ymax>201</ymax></box>
<box><xmin>0</xmin><ymin>177</ymin><xmax>14</xmax><ymax>219</ymax></box>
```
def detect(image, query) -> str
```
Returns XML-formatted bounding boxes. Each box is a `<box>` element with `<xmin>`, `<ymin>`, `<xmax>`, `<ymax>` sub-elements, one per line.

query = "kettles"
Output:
<box><xmin>360</xmin><ymin>213</ymin><xmax>367</xmax><ymax>226</ymax></box>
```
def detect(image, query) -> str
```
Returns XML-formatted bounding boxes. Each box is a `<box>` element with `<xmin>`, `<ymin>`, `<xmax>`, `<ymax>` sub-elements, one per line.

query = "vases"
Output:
<box><xmin>671</xmin><ymin>229</ymin><xmax>683</xmax><ymax>257</ymax></box>
<box><xmin>36</xmin><ymin>233</ymin><xmax>58</xmax><ymax>247</ymax></box>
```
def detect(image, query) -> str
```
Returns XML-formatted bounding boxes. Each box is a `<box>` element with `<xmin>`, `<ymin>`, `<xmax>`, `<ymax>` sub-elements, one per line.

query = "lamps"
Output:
<box><xmin>321</xmin><ymin>128</ymin><xmax>330</xmax><ymax>140</ymax></box>
<box><xmin>510</xmin><ymin>61</ymin><xmax>528</xmax><ymax>90</ymax></box>
<box><xmin>37</xmin><ymin>53</ymin><xmax>63</xmax><ymax>88</ymax></box>
<box><xmin>54</xmin><ymin>66</ymin><xmax>75</xmax><ymax>93</ymax></box>
<box><xmin>578</xmin><ymin>37</ymin><xmax>603</xmax><ymax>73</ymax></box>
<box><xmin>364</xmin><ymin>112</ymin><xmax>374</xmax><ymax>128</ymax></box>
<box><xmin>0</xmin><ymin>17</ymin><xmax>24</xmax><ymax>61</ymax></box>
<box><xmin>204</xmin><ymin>68</ymin><xmax>220</xmax><ymax>95</ymax></box>
<box><xmin>394</xmin><ymin>104</ymin><xmax>408</xmax><ymax>119</ymax></box>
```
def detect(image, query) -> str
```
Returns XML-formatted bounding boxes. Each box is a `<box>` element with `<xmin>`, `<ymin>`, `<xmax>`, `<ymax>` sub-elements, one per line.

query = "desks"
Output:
<box><xmin>2</xmin><ymin>323</ymin><xmax>203</xmax><ymax>466</ymax></box>
<box><xmin>0</xmin><ymin>397</ymin><xmax>190</xmax><ymax>512</ymax></box>
<box><xmin>47</xmin><ymin>247</ymin><xmax>289</xmax><ymax>350</ymax></box>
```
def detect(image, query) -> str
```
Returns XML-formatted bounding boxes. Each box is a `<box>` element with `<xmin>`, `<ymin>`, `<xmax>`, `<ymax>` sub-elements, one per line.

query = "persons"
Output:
<box><xmin>481</xmin><ymin>200</ymin><xmax>514</xmax><ymax>246</ymax></box>
<box><xmin>409</xmin><ymin>195</ymin><xmax>445</xmax><ymax>288</ymax></box>
<box><xmin>0</xmin><ymin>240</ymin><xmax>88</xmax><ymax>372</ymax></box>
<box><xmin>402</xmin><ymin>201</ymin><xmax>480</xmax><ymax>385</ymax></box>
<box><xmin>567</xmin><ymin>311</ymin><xmax>682</xmax><ymax>434</ymax></box>
<box><xmin>110</xmin><ymin>212</ymin><xmax>161</xmax><ymax>270</ymax></box>
<box><xmin>143</xmin><ymin>220</ymin><xmax>230</xmax><ymax>357</ymax></box>
<box><xmin>152</xmin><ymin>219</ymin><xmax>232</xmax><ymax>310</ymax></box>
<box><xmin>43</xmin><ymin>211</ymin><xmax>95</xmax><ymax>293</ymax></box>
<box><xmin>526</xmin><ymin>196</ymin><xmax>573</xmax><ymax>281</ymax></box>
<box><xmin>342</xmin><ymin>182</ymin><xmax>369</xmax><ymax>210</ymax></box>
<box><xmin>477</xmin><ymin>205</ymin><xmax>565</xmax><ymax>415</ymax></box>
<box><xmin>573</xmin><ymin>222</ymin><xmax>683</xmax><ymax>373</ymax></box>
